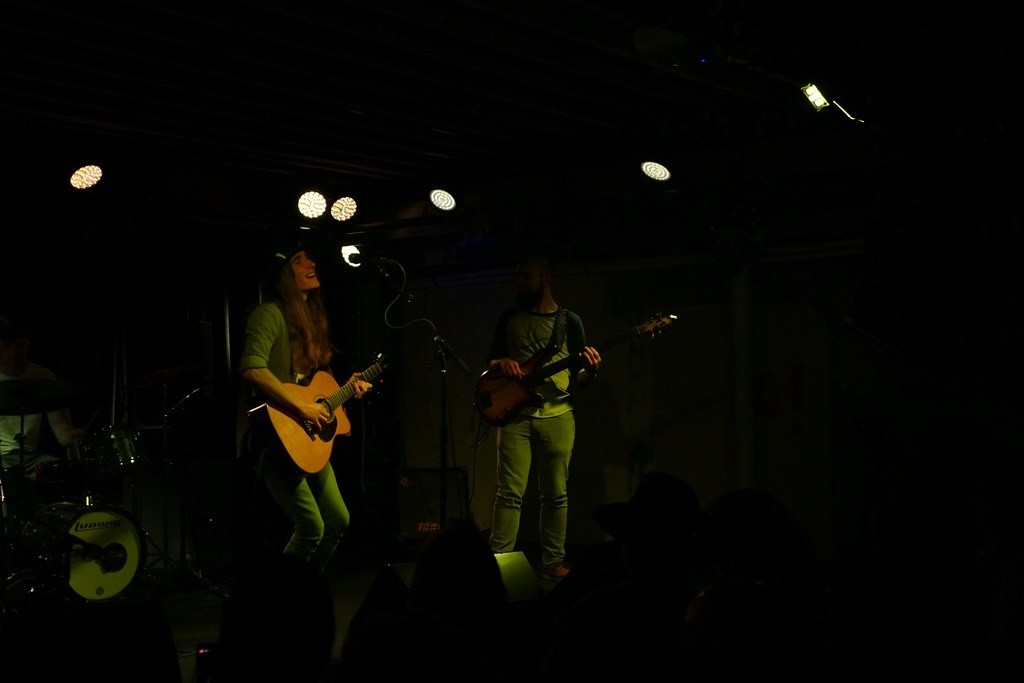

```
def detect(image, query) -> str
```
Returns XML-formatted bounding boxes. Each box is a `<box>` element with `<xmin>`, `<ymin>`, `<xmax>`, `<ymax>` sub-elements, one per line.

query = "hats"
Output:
<box><xmin>253</xmin><ymin>240</ymin><xmax>303</xmax><ymax>297</ymax></box>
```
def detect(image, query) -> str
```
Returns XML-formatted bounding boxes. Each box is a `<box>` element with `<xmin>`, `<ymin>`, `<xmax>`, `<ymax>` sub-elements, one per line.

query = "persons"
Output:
<box><xmin>487</xmin><ymin>256</ymin><xmax>604</xmax><ymax>581</ymax></box>
<box><xmin>0</xmin><ymin>470</ymin><xmax>1024</xmax><ymax>683</ymax></box>
<box><xmin>239</xmin><ymin>241</ymin><xmax>373</xmax><ymax>576</ymax></box>
<box><xmin>1</xmin><ymin>320</ymin><xmax>87</xmax><ymax>526</ymax></box>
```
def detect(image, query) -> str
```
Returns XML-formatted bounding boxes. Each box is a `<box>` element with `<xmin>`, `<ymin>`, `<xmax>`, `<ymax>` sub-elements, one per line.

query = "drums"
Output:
<box><xmin>19</xmin><ymin>497</ymin><xmax>150</xmax><ymax>608</ymax></box>
<box><xmin>72</xmin><ymin>422</ymin><xmax>144</xmax><ymax>474</ymax></box>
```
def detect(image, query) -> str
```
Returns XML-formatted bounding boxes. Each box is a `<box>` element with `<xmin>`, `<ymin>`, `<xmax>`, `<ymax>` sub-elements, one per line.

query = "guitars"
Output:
<box><xmin>249</xmin><ymin>350</ymin><xmax>393</xmax><ymax>476</ymax></box>
<box><xmin>474</xmin><ymin>311</ymin><xmax>678</xmax><ymax>431</ymax></box>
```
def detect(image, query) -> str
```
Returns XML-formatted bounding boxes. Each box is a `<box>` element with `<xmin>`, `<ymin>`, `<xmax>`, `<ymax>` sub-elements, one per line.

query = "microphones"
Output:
<box><xmin>349</xmin><ymin>253</ymin><xmax>388</xmax><ymax>264</ymax></box>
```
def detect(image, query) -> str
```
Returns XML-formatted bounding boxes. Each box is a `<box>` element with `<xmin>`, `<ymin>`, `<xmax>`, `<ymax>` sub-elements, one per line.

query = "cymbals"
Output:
<box><xmin>0</xmin><ymin>376</ymin><xmax>80</xmax><ymax>416</ymax></box>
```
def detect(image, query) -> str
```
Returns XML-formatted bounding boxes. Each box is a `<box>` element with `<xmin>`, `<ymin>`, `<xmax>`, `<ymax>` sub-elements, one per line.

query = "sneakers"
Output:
<box><xmin>543</xmin><ymin>564</ymin><xmax>570</xmax><ymax>580</ymax></box>
